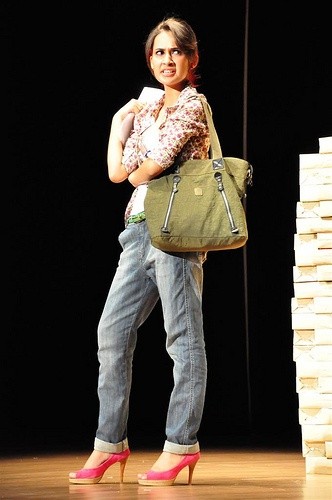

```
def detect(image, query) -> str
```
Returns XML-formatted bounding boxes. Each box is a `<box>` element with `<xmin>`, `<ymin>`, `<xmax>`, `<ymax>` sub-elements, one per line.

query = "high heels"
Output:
<box><xmin>69</xmin><ymin>448</ymin><xmax>131</xmax><ymax>484</ymax></box>
<box><xmin>138</xmin><ymin>451</ymin><xmax>200</xmax><ymax>486</ymax></box>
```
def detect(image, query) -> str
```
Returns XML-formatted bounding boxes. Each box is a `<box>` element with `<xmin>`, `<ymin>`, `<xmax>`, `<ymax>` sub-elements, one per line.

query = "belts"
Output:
<box><xmin>128</xmin><ymin>211</ymin><xmax>146</xmax><ymax>223</ymax></box>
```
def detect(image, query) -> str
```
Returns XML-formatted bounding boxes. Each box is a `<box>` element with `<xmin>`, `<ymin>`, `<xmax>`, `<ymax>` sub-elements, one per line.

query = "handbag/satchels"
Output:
<box><xmin>144</xmin><ymin>96</ymin><xmax>254</xmax><ymax>251</ymax></box>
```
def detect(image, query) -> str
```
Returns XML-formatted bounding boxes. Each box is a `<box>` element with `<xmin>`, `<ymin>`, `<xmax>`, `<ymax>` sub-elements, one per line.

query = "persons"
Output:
<box><xmin>66</xmin><ymin>15</ymin><xmax>217</xmax><ymax>489</ymax></box>
